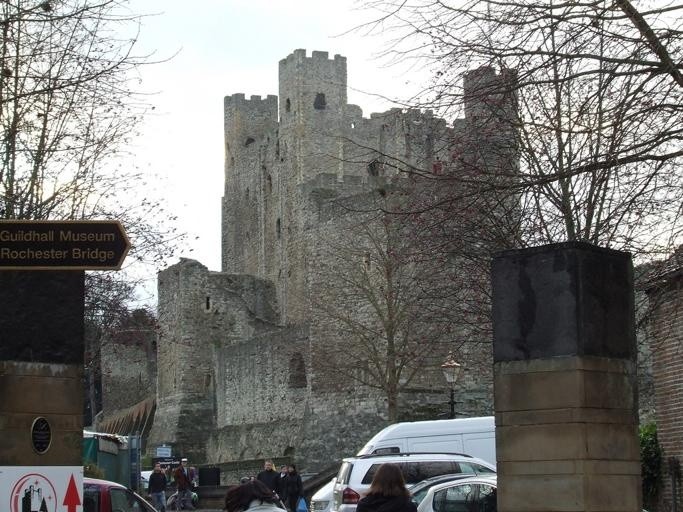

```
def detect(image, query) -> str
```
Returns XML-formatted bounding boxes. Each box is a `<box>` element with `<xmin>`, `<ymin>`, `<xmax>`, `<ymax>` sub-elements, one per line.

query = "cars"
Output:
<box><xmin>399</xmin><ymin>470</ymin><xmax>496</xmax><ymax>510</ymax></box>
<box><xmin>140</xmin><ymin>468</ymin><xmax>197</xmax><ymax>488</ymax></box>
<box><xmin>83</xmin><ymin>477</ymin><xmax>158</xmax><ymax>512</ymax></box>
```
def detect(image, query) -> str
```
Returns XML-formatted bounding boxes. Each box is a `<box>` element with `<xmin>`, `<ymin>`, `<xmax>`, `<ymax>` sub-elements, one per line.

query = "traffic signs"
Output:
<box><xmin>0</xmin><ymin>220</ymin><xmax>130</xmax><ymax>271</ymax></box>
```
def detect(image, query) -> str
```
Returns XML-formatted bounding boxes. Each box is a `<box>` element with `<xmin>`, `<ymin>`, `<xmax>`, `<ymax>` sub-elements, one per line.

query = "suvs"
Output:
<box><xmin>334</xmin><ymin>449</ymin><xmax>496</xmax><ymax>511</ymax></box>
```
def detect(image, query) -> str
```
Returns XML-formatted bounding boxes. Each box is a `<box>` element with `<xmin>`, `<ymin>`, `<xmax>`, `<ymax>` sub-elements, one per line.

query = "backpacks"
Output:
<box><xmin>225</xmin><ymin>480</ymin><xmax>276</xmax><ymax>512</ymax></box>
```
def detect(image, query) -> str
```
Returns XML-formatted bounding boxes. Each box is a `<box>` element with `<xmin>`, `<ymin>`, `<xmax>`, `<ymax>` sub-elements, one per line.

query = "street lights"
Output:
<box><xmin>441</xmin><ymin>348</ymin><xmax>464</xmax><ymax>417</ymax></box>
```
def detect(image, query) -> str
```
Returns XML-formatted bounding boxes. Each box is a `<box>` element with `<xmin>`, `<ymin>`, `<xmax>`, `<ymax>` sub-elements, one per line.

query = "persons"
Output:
<box><xmin>356</xmin><ymin>463</ymin><xmax>417</xmax><ymax>512</ymax></box>
<box><xmin>146</xmin><ymin>461</ymin><xmax>167</xmax><ymax>512</ymax></box>
<box><xmin>222</xmin><ymin>459</ymin><xmax>304</xmax><ymax>512</ymax></box>
<box><xmin>173</xmin><ymin>458</ymin><xmax>195</xmax><ymax>512</ymax></box>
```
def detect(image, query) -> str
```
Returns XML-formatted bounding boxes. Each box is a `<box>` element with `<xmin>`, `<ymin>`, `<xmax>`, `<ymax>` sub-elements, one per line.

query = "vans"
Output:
<box><xmin>308</xmin><ymin>416</ymin><xmax>496</xmax><ymax>511</ymax></box>
